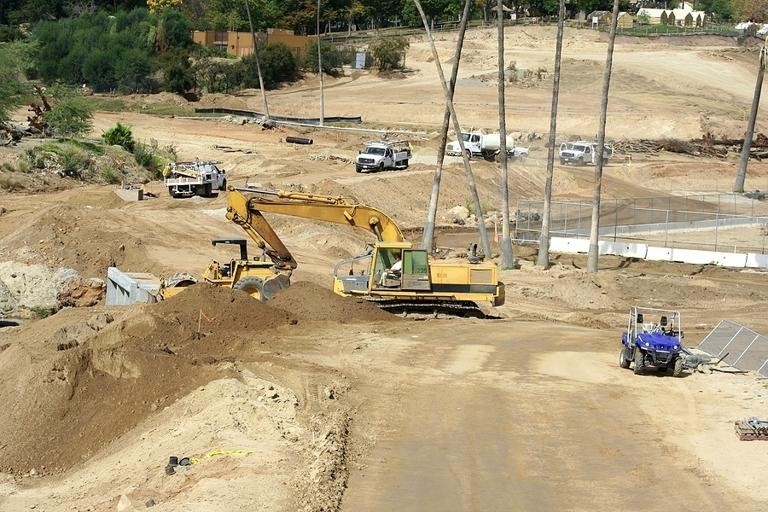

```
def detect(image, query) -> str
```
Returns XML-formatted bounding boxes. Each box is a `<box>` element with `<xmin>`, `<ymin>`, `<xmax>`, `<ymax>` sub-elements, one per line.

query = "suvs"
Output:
<box><xmin>619</xmin><ymin>305</ymin><xmax>684</xmax><ymax>376</ymax></box>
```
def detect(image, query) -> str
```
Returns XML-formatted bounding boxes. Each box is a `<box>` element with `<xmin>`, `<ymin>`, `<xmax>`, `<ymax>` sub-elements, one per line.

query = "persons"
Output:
<box><xmin>375</xmin><ymin>252</ymin><xmax>402</xmax><ymax>286</ymax></box>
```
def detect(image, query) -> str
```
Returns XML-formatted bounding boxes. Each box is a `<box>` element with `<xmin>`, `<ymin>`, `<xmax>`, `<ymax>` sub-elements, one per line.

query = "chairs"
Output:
<box><xmin>384</xmin><ymin>268</ymin><xmax>401</xmax><ymax>287</ymax></box>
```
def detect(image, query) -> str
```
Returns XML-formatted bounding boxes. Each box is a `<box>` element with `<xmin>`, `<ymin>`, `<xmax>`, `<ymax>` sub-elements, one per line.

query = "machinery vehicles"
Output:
<box><xmin>224</xmin><ymin>185</ymin><xmax>505</xmax><ymax>320</ymax></box>
<box><xmin>156</xmin><ymin>239</ymin><xmax>277</xmax><ymax>304</ymax></box>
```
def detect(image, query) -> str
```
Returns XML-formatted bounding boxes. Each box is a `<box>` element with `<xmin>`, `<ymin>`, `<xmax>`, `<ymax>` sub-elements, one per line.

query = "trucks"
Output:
<box><xmin>164</xmin><ymin>163</ymin><xmax>228</xmax><ymax>198</ymax></box>
<box><xmin>355</xmin><ymin>139</ymin><xmax>411</xmax><ymax>173</ymax></box>
<box><xmin>446</xmin><ymin>132</ymin><xmax>514</xmax><ymax>160</ymax></box>
<box><xmin>559</xmin><ymin>141</ymin><xmax>612</xmax><ymax>166</ymax></box>
